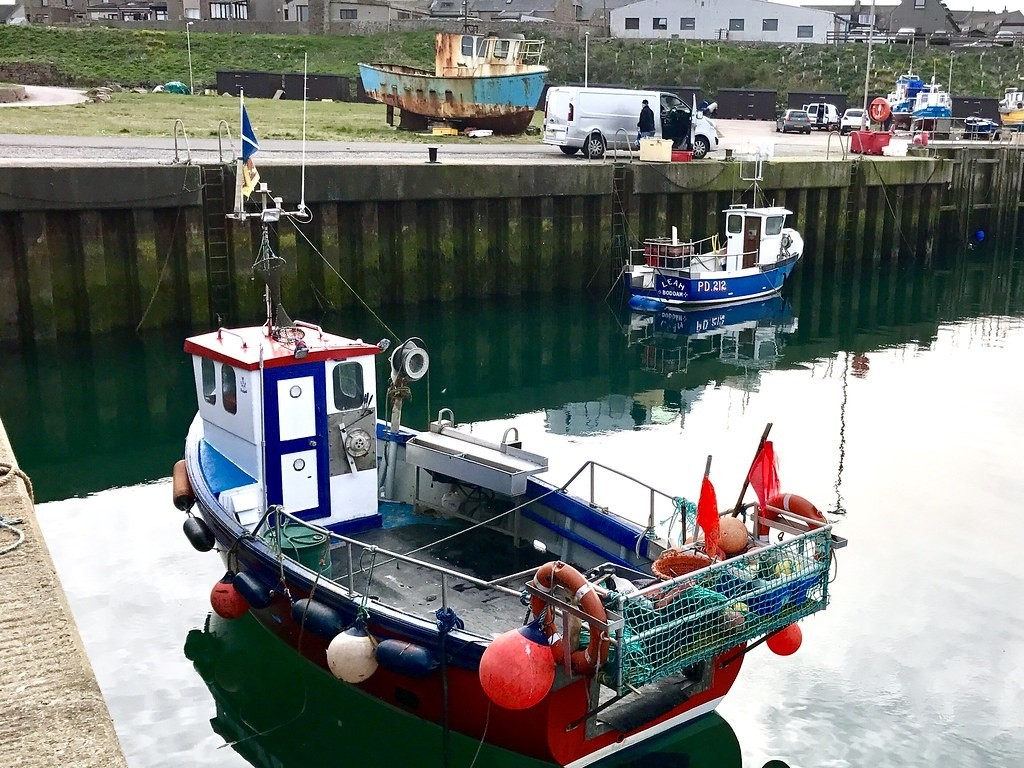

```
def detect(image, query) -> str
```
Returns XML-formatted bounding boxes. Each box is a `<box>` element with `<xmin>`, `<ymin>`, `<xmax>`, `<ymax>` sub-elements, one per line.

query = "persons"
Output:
<box><xmin>637</xmin><ymin>100</ymin><xmax>656</xmax><ymax>138</ymax></box>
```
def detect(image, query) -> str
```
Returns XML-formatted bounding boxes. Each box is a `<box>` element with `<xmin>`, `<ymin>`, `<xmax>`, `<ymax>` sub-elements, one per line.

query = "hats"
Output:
<box><xmin>642</xmin><ymin>100</ymin><xmax>648</xmax><ymax>104</ymax></box>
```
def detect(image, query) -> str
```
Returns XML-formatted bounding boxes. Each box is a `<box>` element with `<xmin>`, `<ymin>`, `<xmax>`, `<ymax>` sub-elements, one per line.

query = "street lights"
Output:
<box><xmin>584</xmin><ymin>32</ymin><xmax>591</xmax><ymax>89</ymax></box>
<box><xmin>186</xmin><ymin>22</ymin><xmax>194</xmax><ymax>95</ymax></box>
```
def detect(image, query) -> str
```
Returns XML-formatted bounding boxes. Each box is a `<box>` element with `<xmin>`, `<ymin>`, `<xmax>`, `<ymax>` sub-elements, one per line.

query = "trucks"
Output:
<box><xmin>545</xmin><ymin>391</ymin><xmax>682</xmax><ymax>436</ymax></box>
<box><xmin>802</xmin><ymin>102</ymin><xmax>842</xmax><ymax>131</ymax></box>
<box><xmin>539</xmin><ymin>86</ymin><xmax>721</xmax><ymax>159</ymax></box>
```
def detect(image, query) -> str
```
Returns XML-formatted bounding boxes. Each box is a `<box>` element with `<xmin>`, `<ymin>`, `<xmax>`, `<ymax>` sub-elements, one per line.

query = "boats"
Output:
<box><xmin>183</xmin><ymin>576</ymin><xmax>741</xmax><ymax>768</ymax></box>
<box><xmin>885</xmin><ymin>74</ymin><xmax>953</xmax><ymax>130</ymax></box>
<box><xmin>623</xmin><ymin>204</ymin><xmax>807</xmax><ymax>305</ymax></box>
<box><xmin>356</xmin><ymin>29</ymin><xmax>552</xmax><ymax>137</ymax></box>
<box><xmin>629</xmin><ymin>293</ymin><xmax>801</xmax><ymax>378</ymax></box>
<box><xmin>998</xmin><ymin>87</ymin><xmax>1024</xmax><ymax>130</ymax></box>
<box><xmin>172</xmin><ymin>87</ymin><xmax>850</xmax><ymax>768</ymax></box>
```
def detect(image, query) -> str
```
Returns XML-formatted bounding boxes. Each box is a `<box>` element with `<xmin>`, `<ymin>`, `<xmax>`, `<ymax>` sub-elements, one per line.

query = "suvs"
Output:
<box><xmin>838</xmin><ymin>108</ymin><xmax>871</xmax><ymax>136</ymax></box>
<box><xmin>775</xmin><ymin>108</ymin><xmax>811</xmax><ymax>134</ymax></box>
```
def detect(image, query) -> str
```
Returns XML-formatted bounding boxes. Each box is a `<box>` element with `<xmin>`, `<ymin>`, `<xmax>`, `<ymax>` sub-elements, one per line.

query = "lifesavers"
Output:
<box><xmin>872</xmin><ymin>102</ymin><xmax>886</xmax><ymax>121</ymax></box>
<box><xmin>531</xmin><ymin>560</ymin><xmax>609</xmax><ymax>673</ymax></box>
<box><xmin>757</xmin><ymin>494</ymin><xmax>832</xmax><ymax>569</ymax></box>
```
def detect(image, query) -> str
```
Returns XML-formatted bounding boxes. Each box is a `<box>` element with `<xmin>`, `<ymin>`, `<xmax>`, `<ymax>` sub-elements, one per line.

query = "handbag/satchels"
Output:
<box><xmin>634</xmin><ymin>132</ymin><xmax>641</xmax><ymax>148</ymax></box>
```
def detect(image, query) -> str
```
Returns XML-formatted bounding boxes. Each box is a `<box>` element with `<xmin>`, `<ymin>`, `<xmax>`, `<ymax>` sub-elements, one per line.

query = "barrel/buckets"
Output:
<box><xmin>263</xmin><ymin>521</ymin><xmax>331</xmax><ymax>582</ymax></box>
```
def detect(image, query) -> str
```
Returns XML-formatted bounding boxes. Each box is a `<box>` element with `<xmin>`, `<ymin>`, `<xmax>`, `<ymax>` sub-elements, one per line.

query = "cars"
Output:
<box><xmin>845</xmin><ymin>26</ymin><xmax>1017</xmax><ymax>45</ymax></box>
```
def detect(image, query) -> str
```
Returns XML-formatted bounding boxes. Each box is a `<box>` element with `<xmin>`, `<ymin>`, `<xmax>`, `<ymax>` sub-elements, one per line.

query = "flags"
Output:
<box><xmin>239</xmin><ymin>89</ymin><xmax>259</xmax><ymax>165</ymax></box>
<box><xmin>696</xmin><ymin>474</ymin><xmax>721</xmax><ymax>560</ymax></box>
<box><xmin>748</xmin><ymin>446</ymin><xmax>780</xmax><ymax>529</ymax></box>
